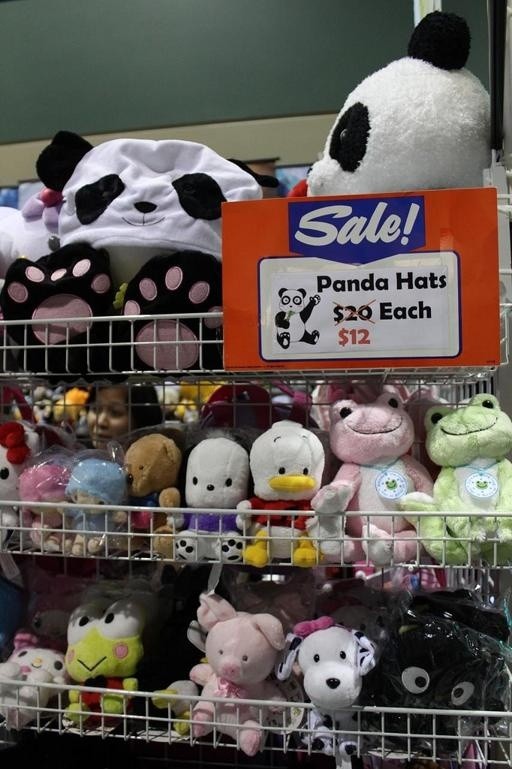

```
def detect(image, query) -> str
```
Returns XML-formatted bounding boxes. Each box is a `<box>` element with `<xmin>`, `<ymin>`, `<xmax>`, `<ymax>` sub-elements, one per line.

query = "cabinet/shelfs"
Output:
<box><xmin>1</xmin><ymin>299</ymin><xmax>512</xmax><ymax>768</ymax></box>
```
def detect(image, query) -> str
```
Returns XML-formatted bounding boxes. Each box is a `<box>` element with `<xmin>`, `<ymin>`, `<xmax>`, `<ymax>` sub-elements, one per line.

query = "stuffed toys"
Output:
<box><xmin>0</xmin><ymin>375</ymin><xmax>511</xmax><ymax>568</ymax></box>
<box><xmin>0</xmin><ymin>9</ymin><xmax>512</xmax><ymax>758</ymax></box>
<box><xmin>1</xmin><ymin>549</ymin><xmax>509</xmax><ymax>759</ymax></box>
<box><xmin>1</xmin><ymin>8</ymin><xmax>493</xmax><ymax>371</ymax></box>
